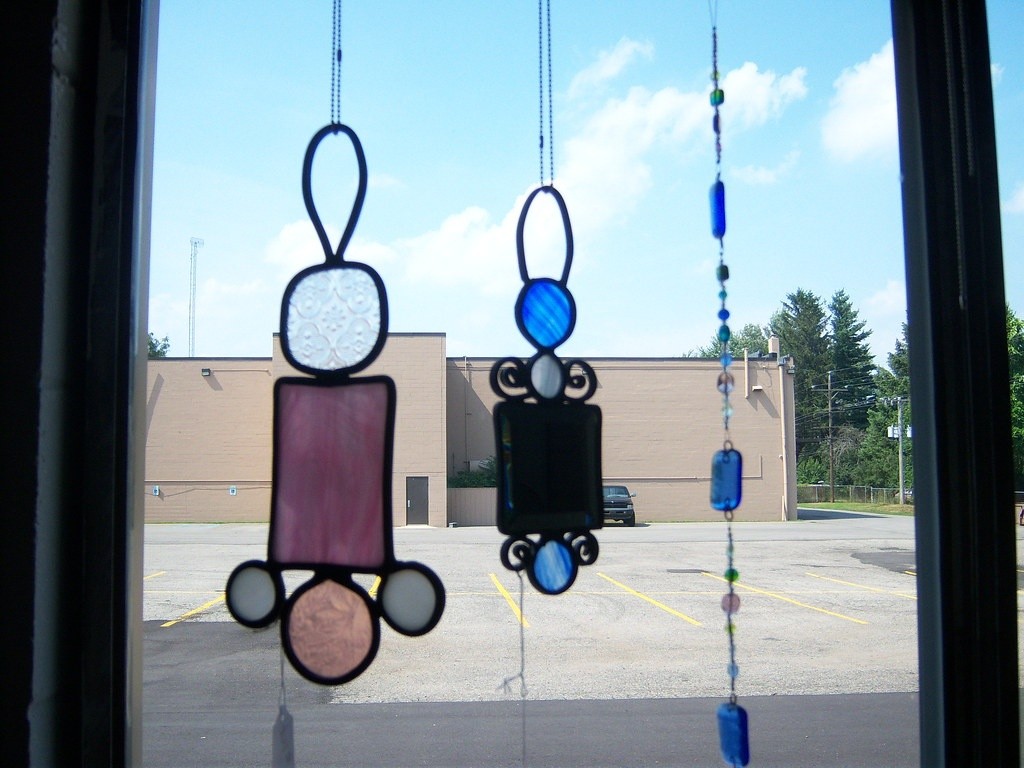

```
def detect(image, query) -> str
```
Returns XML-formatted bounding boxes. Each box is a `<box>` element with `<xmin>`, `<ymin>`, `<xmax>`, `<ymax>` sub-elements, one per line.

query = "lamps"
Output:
<box><xmin>202</xmin><ymin>369</ymin><xmax>210</xmax><ymax>376</ymax></box>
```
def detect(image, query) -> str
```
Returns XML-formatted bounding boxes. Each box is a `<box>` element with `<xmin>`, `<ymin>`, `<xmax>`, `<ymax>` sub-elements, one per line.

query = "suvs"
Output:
<box><xmin>602</xmin><ymin>483</ymin><xmax>636</xmax><ymax>526</ymax></box>
